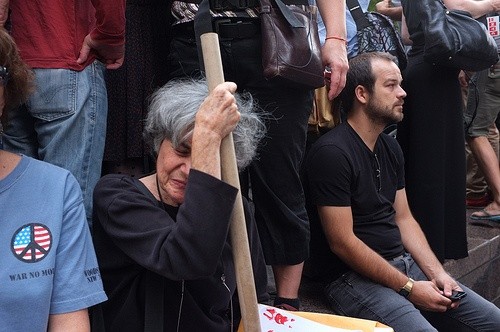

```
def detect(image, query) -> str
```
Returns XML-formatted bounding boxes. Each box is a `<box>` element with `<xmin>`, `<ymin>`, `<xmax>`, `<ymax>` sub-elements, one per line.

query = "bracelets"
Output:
<box><xmin>325</xmin><ymin>37</ymin><xmax>346</xmax><ymax>41</ymax></box>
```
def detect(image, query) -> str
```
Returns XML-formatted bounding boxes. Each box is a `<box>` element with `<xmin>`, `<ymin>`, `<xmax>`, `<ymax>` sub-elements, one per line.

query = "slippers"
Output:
<box><xmin>471</xmin><ymin>209</ymin><xmax>500</xmax><ymax>220</ymax></box>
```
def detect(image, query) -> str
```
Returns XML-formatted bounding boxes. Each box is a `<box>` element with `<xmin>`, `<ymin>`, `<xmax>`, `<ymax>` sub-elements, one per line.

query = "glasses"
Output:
<box><xmin>0</xmin><ymin>67</ymin><xmax>11</xmax><ymax>82</ymax></box>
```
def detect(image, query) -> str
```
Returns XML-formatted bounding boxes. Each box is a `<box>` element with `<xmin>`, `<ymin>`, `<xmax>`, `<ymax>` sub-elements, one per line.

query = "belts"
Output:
<box><xmin>215</xmin><ymin>19</ymin><xmax>258</xmax><ymax>40</ymax></box>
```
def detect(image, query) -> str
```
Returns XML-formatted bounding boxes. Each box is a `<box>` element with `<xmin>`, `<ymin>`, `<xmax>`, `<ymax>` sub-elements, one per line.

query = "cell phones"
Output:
<box><xmin>438</xmin><ymin>287</ymin><xmax>467</xmax><ymax>301</ymax></box>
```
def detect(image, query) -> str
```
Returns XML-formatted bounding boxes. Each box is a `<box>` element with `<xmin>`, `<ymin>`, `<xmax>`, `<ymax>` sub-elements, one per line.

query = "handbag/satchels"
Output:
<box><xmin>260</xmin><ymin>0</ymin><xmax>326</xmax><ymax>88</ymax></box>
<box><xmin>424</xmin><ymin>0</ymin><xmax>498</xmax><ymax>70</ymax></box>
<box><xmin>306</xmin><ymin>83</ymin><xmax>335</xmax><ymax>130</ymax></box>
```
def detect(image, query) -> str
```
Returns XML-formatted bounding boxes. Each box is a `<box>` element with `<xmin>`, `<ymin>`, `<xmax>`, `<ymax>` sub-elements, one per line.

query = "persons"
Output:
<box><xmin>301</xmin><ymin>52</ymin><xmax>500</xmax><ymax>332</ymax></box>
<box><xmin>90</xmin><ymin>80</ymin><xmax>270</xmax><ymax>332</ymax></box>
<box><xmin>0</xmin><ymin>30</ymin><xmax>108</xmax><ymax>332</ymax></box>
<box><xmin>169</xmin><ymin>0</ymin><xmax>350</xmax><ymax>310</ymax></box>
<box><xmin>0</xmin><ymin>0</ymin><xmax>126</xmax><ymax>222</ymax></box>
<box><xmin>317</xmin><ymin>0</ymin><xmax>500</xmax><ymax>263</ymax></box>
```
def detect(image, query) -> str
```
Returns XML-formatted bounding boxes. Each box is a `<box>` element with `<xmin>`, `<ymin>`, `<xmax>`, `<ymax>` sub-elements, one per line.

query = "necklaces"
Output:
<box><xmin>157</xmin><ymin>175</ymin><xmax>168</xmax><ymax>211</ymax></box>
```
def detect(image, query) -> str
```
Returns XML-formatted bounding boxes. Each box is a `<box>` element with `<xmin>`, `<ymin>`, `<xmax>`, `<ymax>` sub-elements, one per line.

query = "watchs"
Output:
<box><xmin>399</xmin><ymin>278</ymin><xmax>414</xmax><ymax>297</ymax></box>
